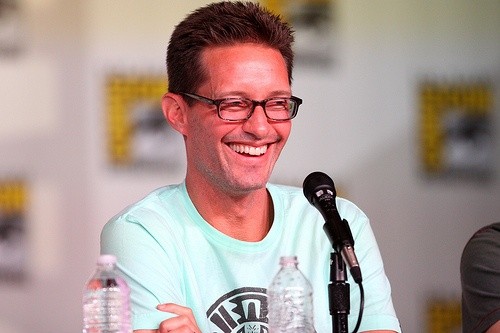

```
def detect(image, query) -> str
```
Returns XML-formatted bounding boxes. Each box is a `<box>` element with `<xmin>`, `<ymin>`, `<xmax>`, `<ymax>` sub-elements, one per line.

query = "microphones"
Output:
<box><xmin>303</xmin><ymin>171</ymin><xmax>362</xmax><ymax>283</ymax></box>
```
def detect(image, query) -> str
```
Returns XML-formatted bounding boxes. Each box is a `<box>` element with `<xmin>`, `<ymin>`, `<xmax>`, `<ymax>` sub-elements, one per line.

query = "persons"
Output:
<box><xmin>460</xmin><ymin>222</ymin><xmax>500</xmax><ymax>333</ymax></box>
<box><xmin>100</xmin><ymin>0</ymin><xmax>403</xmax><ymax>333</ymax></box>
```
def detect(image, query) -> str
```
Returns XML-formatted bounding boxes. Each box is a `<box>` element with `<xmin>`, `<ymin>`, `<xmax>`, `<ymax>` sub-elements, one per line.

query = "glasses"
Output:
<box><xmin>177</xmin><ymin>88</ymin><xmax>302</xmax><ymax>122</ymax></box>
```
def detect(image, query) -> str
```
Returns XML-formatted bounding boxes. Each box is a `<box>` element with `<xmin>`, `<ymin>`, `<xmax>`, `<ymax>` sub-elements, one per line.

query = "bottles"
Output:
<box><xmin>80</xmin><ymin>254</ymin><xmax>132</xmax><ymax>333</ymax></box>
<box><xmin>266</xmin><ymin>255</ymin><xmax>318</xmax><ymax>333</ymax></box>
